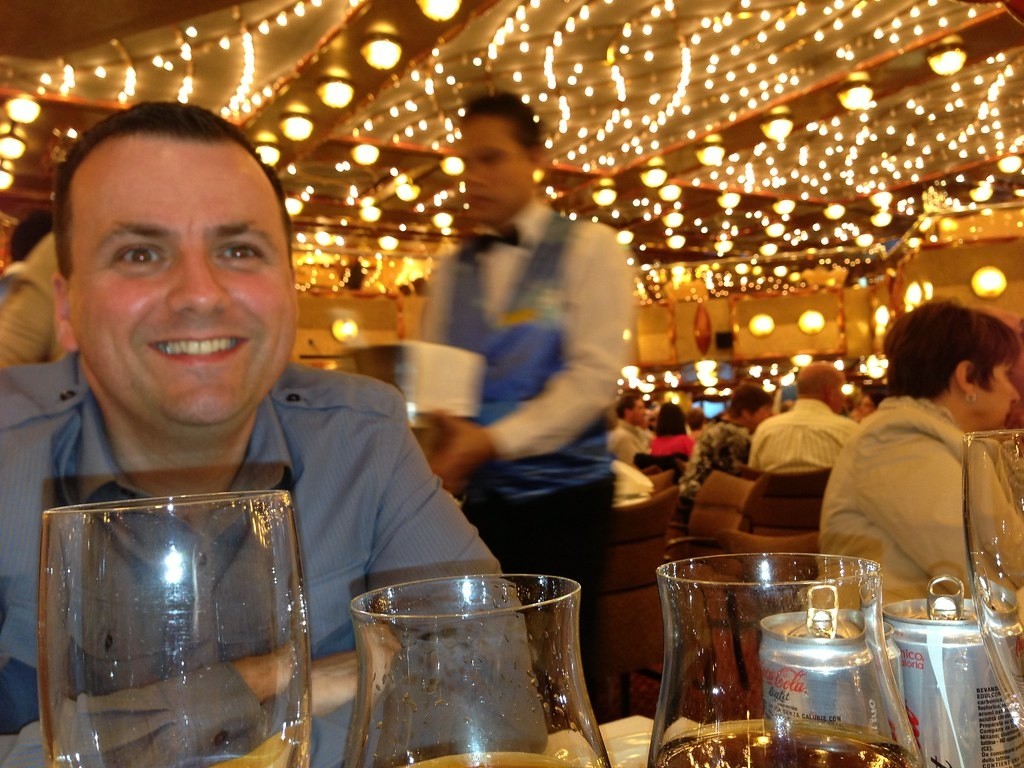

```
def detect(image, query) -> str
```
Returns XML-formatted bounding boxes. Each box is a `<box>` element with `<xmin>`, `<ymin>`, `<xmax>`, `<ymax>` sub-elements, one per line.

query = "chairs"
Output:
<box><xmin>603</xmin><ymin>453</ymin><xmax>839</xmax><ymax>722</ymax></box>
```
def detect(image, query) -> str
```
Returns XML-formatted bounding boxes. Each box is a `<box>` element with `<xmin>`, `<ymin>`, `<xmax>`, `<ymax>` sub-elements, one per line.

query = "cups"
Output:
<box><xmin>963</xmin><ymin>426</ymin><xmax>1023</xmax><ymax>744</ymax></box>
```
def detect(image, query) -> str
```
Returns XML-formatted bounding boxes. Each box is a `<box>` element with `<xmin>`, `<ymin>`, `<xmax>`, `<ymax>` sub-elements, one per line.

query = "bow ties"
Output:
<box><xmin>466</xmin><ymin>223</ymin><xmax>523</xmax><ymax>252</ymax></box>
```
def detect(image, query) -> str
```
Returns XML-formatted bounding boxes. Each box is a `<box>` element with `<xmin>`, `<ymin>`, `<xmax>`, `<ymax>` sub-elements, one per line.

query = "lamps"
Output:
<box><xmin>0</xmin><ymin>96</ymin><xmax>39</xmax><ymax>191</ymax></box>
<box><xmin>256</xmin><ymin>0</ymin><xmax>1022</xmax><ymax>277</ymax></box>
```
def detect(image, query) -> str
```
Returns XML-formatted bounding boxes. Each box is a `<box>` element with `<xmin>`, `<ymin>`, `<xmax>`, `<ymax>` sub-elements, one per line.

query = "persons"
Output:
<box><xmin>0</xmin><ymin>100</ymin><xmax>549</xmax><ymax>768</ymax></box>
<box><xmin>814</xmin><ymin>296</ymin><xmax>1024</xmax><ymax>611</ymax></box>
<box><xmin>405</xmin><ymin>91</ymin><xmax>637</xmax><ymax>726</ymax></box>
<box><xmin>666</xmin><ymin>364</ymin><xmax>888</xmax><ymax>543</ymax></box>
<box><xmin>0</xmin><ymin>212</ymin><xmax>64</xmax><ymax>367</ymax></box>
<box><xmin>605</xmin><ymin>394</ymin><xmax>707</xmax><ymax>465</ymax></box>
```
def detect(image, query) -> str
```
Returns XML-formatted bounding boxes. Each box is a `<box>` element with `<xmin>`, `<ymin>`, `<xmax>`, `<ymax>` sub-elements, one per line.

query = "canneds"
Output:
<box><xmin>875</xmin><ymin>572</ymin><xmax>1024</xmax><ymax>768</ymax></box>
<box><xmin>759</xmin><ymin>584</ymin><xmax>907</xmax><ymax>756</ymax></box>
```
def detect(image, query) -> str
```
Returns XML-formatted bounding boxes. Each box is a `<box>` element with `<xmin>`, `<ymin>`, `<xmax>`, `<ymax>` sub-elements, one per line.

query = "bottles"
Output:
<box><xmin>649</xmin><ymin>551</ymin><xmax>929</xmax><ymax>768</ymax></box>
<box><xmin>38</xmin><ymin>491</ymin><xmax>314</xmax><ymax>767</ymax></box>
<box><xmin>339</xmin><ymin>570</ymin><xmax>610</xmax><ymax>767</ymax></box>
